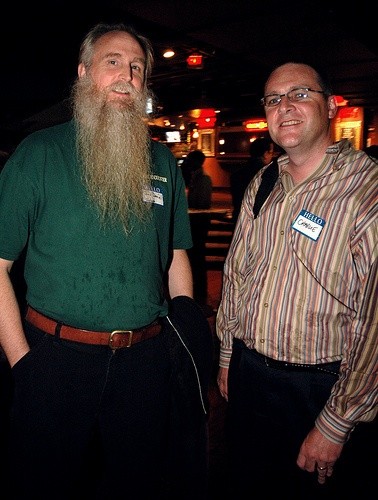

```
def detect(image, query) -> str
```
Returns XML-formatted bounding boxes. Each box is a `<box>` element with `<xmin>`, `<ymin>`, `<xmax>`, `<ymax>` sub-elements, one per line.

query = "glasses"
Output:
<box><xmin>260</xmin><ymin>87</ymin><xmax>324</xmax><ymax>108</ymax></box>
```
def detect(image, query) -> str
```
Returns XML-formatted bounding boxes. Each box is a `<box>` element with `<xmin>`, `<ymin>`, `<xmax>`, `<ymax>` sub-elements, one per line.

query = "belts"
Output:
<box><xmin>23</xmin><ymin>306</ymin><xmax>162</xmax><ymax>350</ymax></box>
<box><xmin>248</xmin><ymin>347</ymin><xmax>343</xmax><ymax>378</ymax></box>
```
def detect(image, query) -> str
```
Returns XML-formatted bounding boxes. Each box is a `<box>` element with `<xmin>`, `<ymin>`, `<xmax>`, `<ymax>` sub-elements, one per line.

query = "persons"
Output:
<box><xmin>215</xmin><ymin>61</ymin><xmax>378</xmax><ymax>500</ymax></box>
<box><xmin>0</xmin><ymin>24</ymin><xmax>215</xmax><ymax>500</ymax></box>
<box><xmin>180</xmin><ymin>150</ymin><xmax>213</xmax><ymax>318</ymax></box>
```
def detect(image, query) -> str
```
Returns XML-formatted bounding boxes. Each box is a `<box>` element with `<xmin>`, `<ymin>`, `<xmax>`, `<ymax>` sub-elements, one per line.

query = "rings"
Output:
<box><xmin>317</xmin><ymin>465</ymin><xmax>327</xmax><ymax>470</ymax></box>
